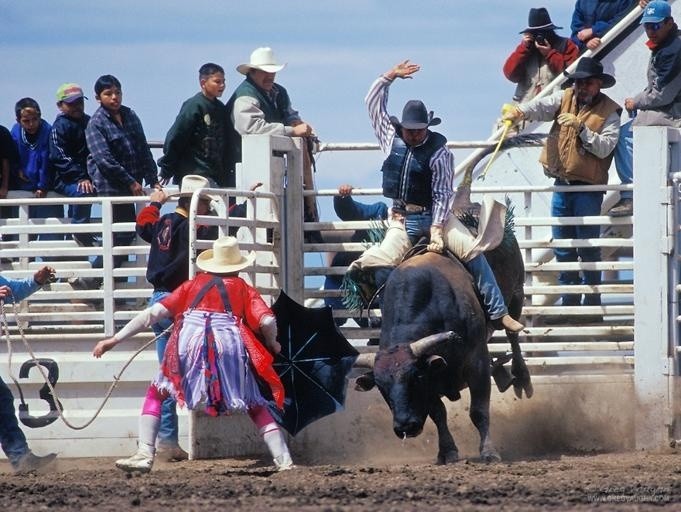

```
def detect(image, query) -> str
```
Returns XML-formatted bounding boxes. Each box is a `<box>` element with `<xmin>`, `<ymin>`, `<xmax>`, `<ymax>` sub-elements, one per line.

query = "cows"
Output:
<box><xmin>353</xmin><ymin>132</ymin><xmax>550</xmax><ymax>466</ymax></box>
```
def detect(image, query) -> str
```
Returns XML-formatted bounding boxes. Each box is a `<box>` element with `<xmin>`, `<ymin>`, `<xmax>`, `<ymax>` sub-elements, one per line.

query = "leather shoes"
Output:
<box><xmin>11</xmin><ymin>452</ymin><xmax>57</xmax><ymax>474</ymax></box>
<box><xmin>497</xmin><ymin>315</ymin><xmax>524</xmax><ymax>334</ymax></box>
<box><xmin>113</xmin><ymin>445</ymin><xmax>155</xmax><ymax>473</ymax></box>
<box><xmin>273</xmin><ymin>453</ymin><xmax>298</xmax><ymax>471</ymax></box>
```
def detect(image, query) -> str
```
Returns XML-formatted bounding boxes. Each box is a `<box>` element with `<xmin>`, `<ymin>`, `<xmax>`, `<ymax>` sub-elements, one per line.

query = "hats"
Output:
<box><xmin>563</xmin><ymin>56</ymin><xmax>616</xmax><ymax>88</ymax></box>
<box><xmin>235</xmin><ymin>47</ymin><xmax>285</xmax><ymax>76</ymax></box>
<box><xmin>195</xmin><ymin>235</ymin><xmax>254</xmax><ymax>275</ymax></box>
<box><xmin>519</xmin><ymin>7</ymin><xmax>564</xmax><ymax>33</ymax></box>
<box><xmin>638</xmin><ymin>0</ymin><xmax>672</xmax><ymax>24</ymax></box>
<box><xmin>168</xmin><ymin>174</ymin><xmax>216</xmax><ymax>201</ymax></box>
<box><xmin>55</xmin><ymin>83</ymin><xmax>88</xmax><ymax>104</ymax></box>
<box><xmin>390</xmin><ymin>98</ymin><xmax>441</xmax><ymax>131</ymax></box>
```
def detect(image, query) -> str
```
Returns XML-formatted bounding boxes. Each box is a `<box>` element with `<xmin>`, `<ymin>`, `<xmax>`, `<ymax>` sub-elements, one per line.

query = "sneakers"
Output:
<box><xmin>607</xmin><ymin>198</ymin><xmax>633</xmax><ymax>217</ymax></box>
<box><xmin>154</xmin><ymin>445</ymin><xmax>189</xmax><ymax>461</ymax></box>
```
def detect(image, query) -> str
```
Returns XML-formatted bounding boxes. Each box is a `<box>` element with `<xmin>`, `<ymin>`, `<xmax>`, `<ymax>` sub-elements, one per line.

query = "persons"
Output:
<box><xmin>84</xmin><ymin>75</ymin><xmax>162</xmax><ymax>308</ymax></box>
<box><xmin>504</xmin><ymin>8</ymin><xmax>582</xmax><ymax>105</ymax></box>
<box><xmin>570</xmin><ymin>1</ymin><xmax>652</xmax><ymax>57</ymax></box>
<box><xmin>9</xmin><ymin>98</ymin><xmax>65</xmax><ymax>261</ymax></box>
<box><xmin>154</xmin><ymin>62</ymin><xmax>234</xmax><ymax>189</ymax></box>
<box><xmin>92</xmin><ymin>236</ymin><xmax>299</xmax><ymax>470</ymax></box>
<box><xmin>0</xmin><ymin>266</ymin><xmax>58</xmax><ymax>475</ymax></box>
<box><xmin>47</xmin><ymin>82</ymin><xmax>95</xmax><ymax>250</ymax></box>
<box><xmin>611</xmin><ymin>0</ymin><xmax>681</xmax><ymax>216</ymax></box>
<box><xmin>1</xmin><ymin>125</ymin><xmax>19</xmax><ymax>199</ymax></box>
<box><xmin>511</xmin><ymin>58</ymin><xmax>625</xmax><ymax>325</ymax></box>
<box><xmin>228</xmin><ymin>47</ymin><xmax>321</xmax><ymax>242</ymax></box>
<box><xmin>357</xmin><ymin>59</ymin><xmax>526</xmax><ymax>390</ymax></box>
<box><xmin>323</xmin><ymin>184</ymin><xmax>396</xmax><ymax>330</ymax></box>
<box><xmin>137</xmin><ymin>175</ymin><xmax>264</xmax><ymax>460</ymax></box>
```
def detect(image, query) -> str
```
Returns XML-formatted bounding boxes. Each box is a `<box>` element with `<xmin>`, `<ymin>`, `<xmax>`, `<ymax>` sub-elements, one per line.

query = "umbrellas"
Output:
<box><xmin>247</xmin><ymin>289</ymin><xmax>360</xmax><ymax>435</ymax></box>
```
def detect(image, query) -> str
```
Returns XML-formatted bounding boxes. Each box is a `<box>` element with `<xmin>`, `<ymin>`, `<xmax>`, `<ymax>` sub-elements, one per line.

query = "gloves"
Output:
<box><xmin>557</xmin><ymin>112</ymin><xmax>581</xmax><ymax>130</ymax></box>
<box><xmin>502</xmin><ymin>106</ymin><xmax>521</xmax><ymax>124</ymax></box>
<box><xmin>427</xmin><ymin>226</ymin><xmax>444</xmax><ymax>253</ymax></box>
